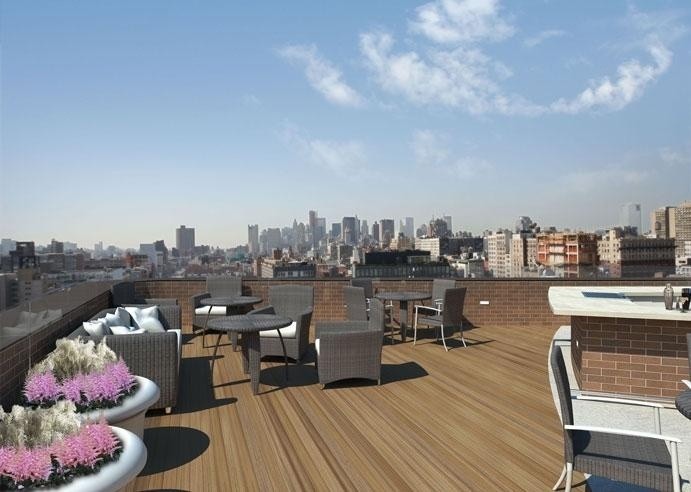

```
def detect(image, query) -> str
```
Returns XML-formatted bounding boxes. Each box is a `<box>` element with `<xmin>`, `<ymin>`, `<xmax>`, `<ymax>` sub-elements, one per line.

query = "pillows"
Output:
<box><xmin>83</xmin><ymin>305</ymin><xmax>165</xmax><ymax>336</ymax></box>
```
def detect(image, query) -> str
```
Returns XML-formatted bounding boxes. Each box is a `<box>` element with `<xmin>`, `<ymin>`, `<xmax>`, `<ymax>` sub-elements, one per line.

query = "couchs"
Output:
<box><xmin>66</xmin><ymin>306</ymin><xmax>182</xmax><ymax>414</ymax></box>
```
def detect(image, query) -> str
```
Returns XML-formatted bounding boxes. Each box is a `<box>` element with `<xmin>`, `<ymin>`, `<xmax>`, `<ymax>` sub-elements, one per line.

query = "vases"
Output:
<box><xmin>36</xmin><ymin>424</ymin><xmax>147</xmax><ymax>492</ymax></box>
<box><xmin>68</xmin><ymin>374</ymin><xmax>160</xmax><ymax>442</ymax></box>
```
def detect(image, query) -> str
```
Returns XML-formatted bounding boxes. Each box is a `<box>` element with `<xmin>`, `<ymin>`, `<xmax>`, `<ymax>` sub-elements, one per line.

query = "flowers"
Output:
<box><xmin>0</xmin><ymin>400</ymin><xmax>125</xmax><ymax>492</ymax></box>
<box><xmin>21</xmin><ymin>336</ymin><xmax>141</xmax><ymax>413</ymax></box>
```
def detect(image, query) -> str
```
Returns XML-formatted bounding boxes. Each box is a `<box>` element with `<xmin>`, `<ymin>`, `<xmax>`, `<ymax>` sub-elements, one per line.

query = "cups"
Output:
<box><xmin>681</xmin><ymin>288</ymin><xmax>691</xmax><ymax>309</ymax></box>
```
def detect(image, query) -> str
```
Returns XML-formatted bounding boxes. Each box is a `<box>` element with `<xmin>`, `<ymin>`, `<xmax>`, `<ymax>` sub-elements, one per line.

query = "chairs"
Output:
<box><xmin>344</xmin><ymin>278</ymin><xmax>467</xmax><ymax>352</ymax></box>
<box><xmin>190</xmin><ymin>275</ymin><xmax>384</xmax><ymax>395</ymax></box>
<box><xmin>547</xmin><ymin>345</ymin><xmax>684</xmax><ymax>492</ymax></box>
<box><xmin>111</xmin><ymin>281</ymin><xmax>178</xmax><ymax>306</ymax></box>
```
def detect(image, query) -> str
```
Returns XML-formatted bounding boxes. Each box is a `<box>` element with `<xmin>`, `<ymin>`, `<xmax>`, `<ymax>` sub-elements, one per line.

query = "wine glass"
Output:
<box><xmin>677</xmin><ymin>298</ymin><xmax>687</xmax><ymax>313</ymax></box>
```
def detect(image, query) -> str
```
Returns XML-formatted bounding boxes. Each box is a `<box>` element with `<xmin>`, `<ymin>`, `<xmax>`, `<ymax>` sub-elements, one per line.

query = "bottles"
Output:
<box><xmin>664</xmin><ymin>283</ymin><xmax>674</xmax><ymax>310</ymax></box>
<box><xmin>676</xmin><ymin>302</ymin><xmax>683</xmax><ymax>311</ymax></box>
